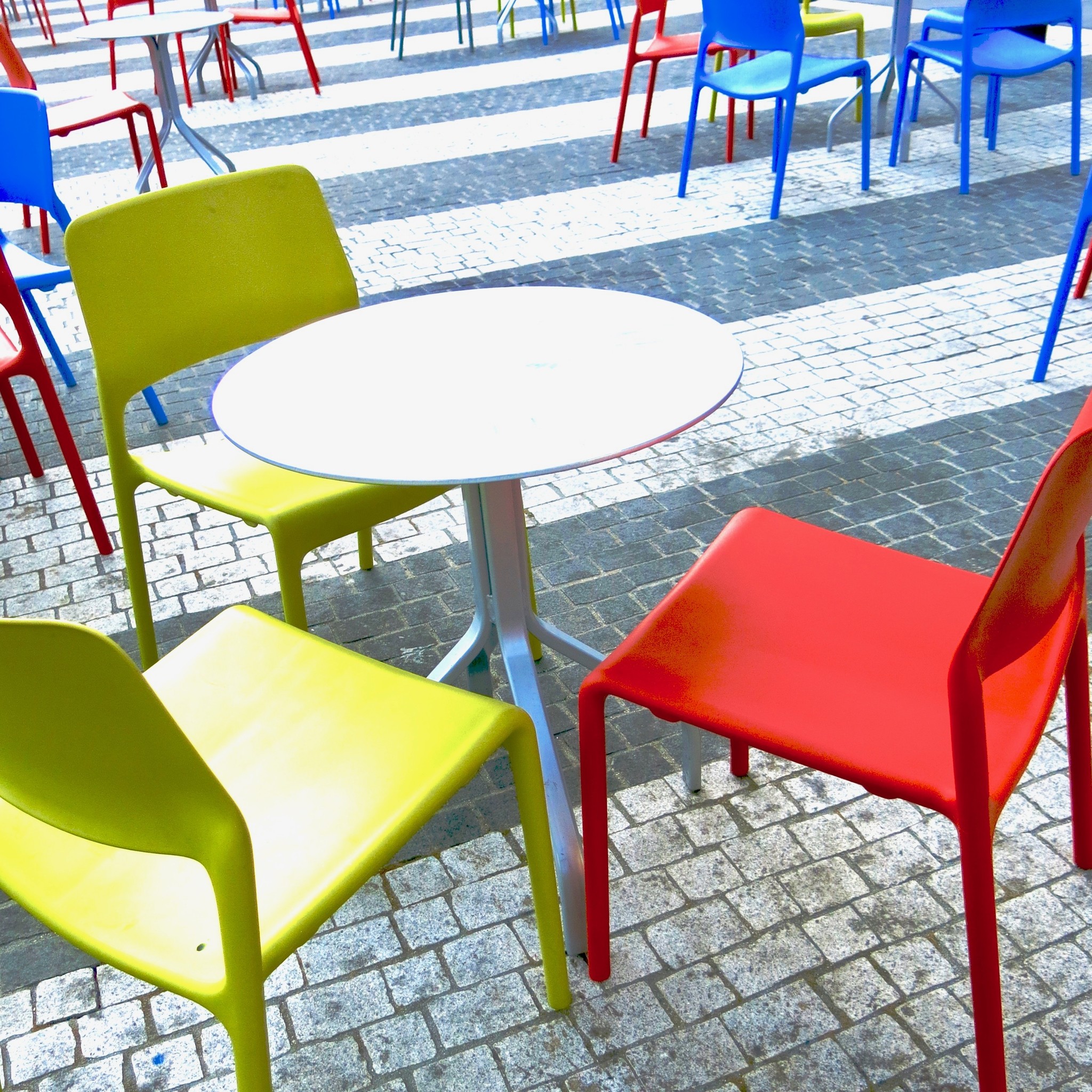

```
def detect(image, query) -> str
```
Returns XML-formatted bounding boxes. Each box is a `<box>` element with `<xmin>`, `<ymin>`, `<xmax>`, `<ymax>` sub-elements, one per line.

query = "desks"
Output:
<box><xmin>210</xmin><ymin>287</ymin><xmax>744</xmax><ymax>953</ymax></box>
<box><xmin>69</xmin><ymin>11</ymin><xmax>239</xmax><ymax>190</ymax></box>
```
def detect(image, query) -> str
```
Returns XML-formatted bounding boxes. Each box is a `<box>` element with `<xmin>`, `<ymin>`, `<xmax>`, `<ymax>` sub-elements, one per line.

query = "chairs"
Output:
<box><xmin>0</xmin><ymin>0</ymin><xmax>1092</xmax><ymax>1091</ymax></box>
